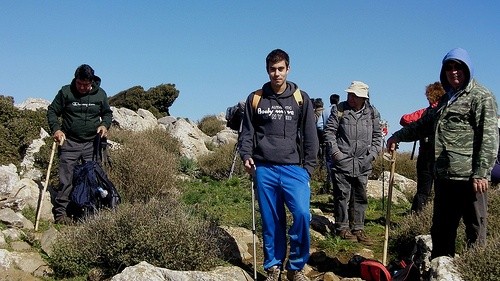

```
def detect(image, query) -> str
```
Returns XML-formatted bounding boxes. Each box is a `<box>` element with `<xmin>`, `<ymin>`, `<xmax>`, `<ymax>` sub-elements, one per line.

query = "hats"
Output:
<box><xmin>344</xmin><ymin>80</ymin><xmax>370</xmax><ymax>99</ymax></box>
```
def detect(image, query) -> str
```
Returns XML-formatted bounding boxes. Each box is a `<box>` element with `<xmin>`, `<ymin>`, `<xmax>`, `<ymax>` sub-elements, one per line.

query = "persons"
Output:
<box><xmin>236</xmin><ymin>49</ymin><xmax>320</xmax><ymax>281</ymax></box>
<box><xmin>399</xmin><ymin>78</ymin><xmax>446</xmax><ymax>213</ymax></box>
<box><xmin>387</xmin><ymin>47</ymin><xmax>500</xmax><ymax>263</ymax></box>
<box><xmin>323</xmin><ymin>81</ymin><xmax>381</xmax><ymax>246</ymax></box>
<box><xmin>308</xmin><ymin>93</ymin><xmax>342</xmax><ymax>195</ymax></box>
<box><xmin>45</xmin><ymin>63</ymin><xmax>113</xmax><ymax>227</ymax></box>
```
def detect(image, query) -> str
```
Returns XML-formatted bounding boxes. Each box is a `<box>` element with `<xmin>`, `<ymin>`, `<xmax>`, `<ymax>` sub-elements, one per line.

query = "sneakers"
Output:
<box><xmin>287</xmin><ymin>271</ymin><xmax>310</xmax><ymax>281</ymax></box>
<box><xmin>265</xmin><ymin>266</ymin><xmax>282</xmax><ymax>281</ymax></box>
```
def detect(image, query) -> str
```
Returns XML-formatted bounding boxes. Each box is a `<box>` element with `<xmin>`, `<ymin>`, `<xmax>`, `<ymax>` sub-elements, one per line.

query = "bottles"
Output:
<box><xmin>98</xmin><ymin>188</ymin><xmax>107</xmax><ymax>198</ymax></box>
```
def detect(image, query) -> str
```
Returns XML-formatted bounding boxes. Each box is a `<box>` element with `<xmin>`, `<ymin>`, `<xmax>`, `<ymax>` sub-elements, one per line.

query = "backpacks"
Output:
<box><xmin>72</xmin><ymin>132</ymin><xmax>121</xmax><ymax>214</ymax></box>
<box><xmin>349</xmin><ymin>255</ymin><xmax>420</xmax><ymax>281</ymax></box>
<box><xmin>225</xmin><ymin>104</ymin><xmax>240</xmax><ymax>131</ymax></box>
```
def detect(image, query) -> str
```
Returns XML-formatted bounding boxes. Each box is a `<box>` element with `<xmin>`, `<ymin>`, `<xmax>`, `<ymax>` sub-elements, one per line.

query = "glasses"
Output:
<box><xmin>445</xmin><ymin>65</ymin><xmax>463</xmax><ymax>71</ymax></box>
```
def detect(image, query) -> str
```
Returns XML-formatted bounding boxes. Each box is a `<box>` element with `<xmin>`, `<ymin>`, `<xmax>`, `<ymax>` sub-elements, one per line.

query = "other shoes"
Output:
<box><xmin>352</xmin><ymin>230</ymin><xmax>373</xmax><ymax>245</ymax></box>
<box><xmin>336</xmin><ymin>229</ymin><xmax>357</xmax><ymax>241</ymax></box>
